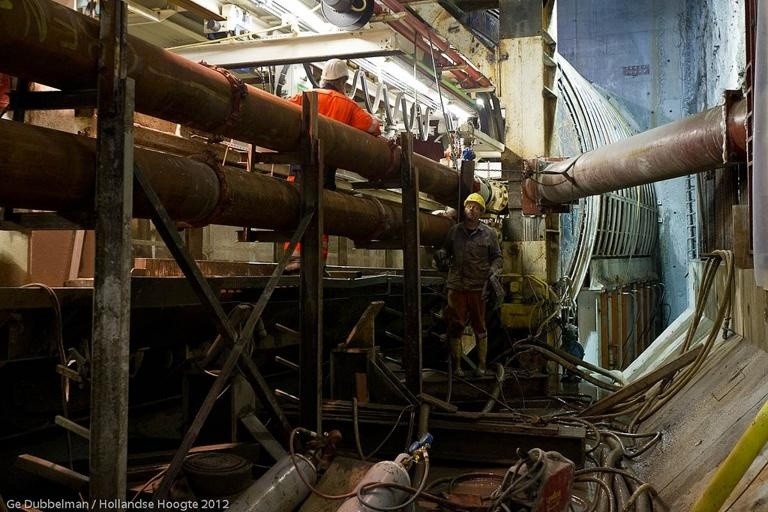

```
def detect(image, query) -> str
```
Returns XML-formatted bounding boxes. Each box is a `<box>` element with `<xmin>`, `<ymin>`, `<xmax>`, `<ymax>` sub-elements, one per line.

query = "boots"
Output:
<box><xmin>449</xmin><ymin>338</ymin><xmax>465</xmax><ymax>377</ymax></box>
<box><xmin>473</xmin><ymin>337</ymin><xmax>487</xmax><ymax>376</ymax></box>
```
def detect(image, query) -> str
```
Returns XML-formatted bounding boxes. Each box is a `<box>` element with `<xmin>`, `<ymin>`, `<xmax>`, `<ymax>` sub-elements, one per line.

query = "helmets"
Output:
<box><xmin>464</xmin><ymin>192</ymin><xmax>485</xmax><ymax>213</ymax></box>
<box><xmin>321</xmin><ymin>58</ymin><xmax>349</xmax><ymax>80</ymax></box>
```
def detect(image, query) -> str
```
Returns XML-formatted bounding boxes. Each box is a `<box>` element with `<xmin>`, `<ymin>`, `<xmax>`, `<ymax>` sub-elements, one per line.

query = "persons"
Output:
<box><xmin>432</xmin><ymin>206</ymin><xmax>456</xmax><ymax>221</ymax></box>
<box><xmin>461</xmin><ymin>139</ymin><xmax>476</xmax><ymax>160</ymax></box>
<box><xmin>434</xmin><ymin>190</ymin><xmax>504</xmax><ymax>377</ymax></box>
<box><xmin>558</xmin><ymin>322</ymin><xmax>587</xmax><ymax>390</ymax></box>
<box><xmin>436</xmin><ymin>142</ymin><xmax>458</xmax><ymax>170</ymax></box>
<box><xmin>280</xmin><ymin>56</ymin><xmax>381</xmax><ymax>273</ymax></box>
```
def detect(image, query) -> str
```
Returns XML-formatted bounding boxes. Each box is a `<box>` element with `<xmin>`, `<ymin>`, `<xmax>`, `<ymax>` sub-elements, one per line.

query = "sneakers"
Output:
<box><xmin>324</xmin><ymin>266</ymin><xmax>331</xmax><ymax>277</ymax></box>
<box><xmin>284</xmin><ymin>259</ymin><xmax>299</xmax><ymax>274</ymax></box>
